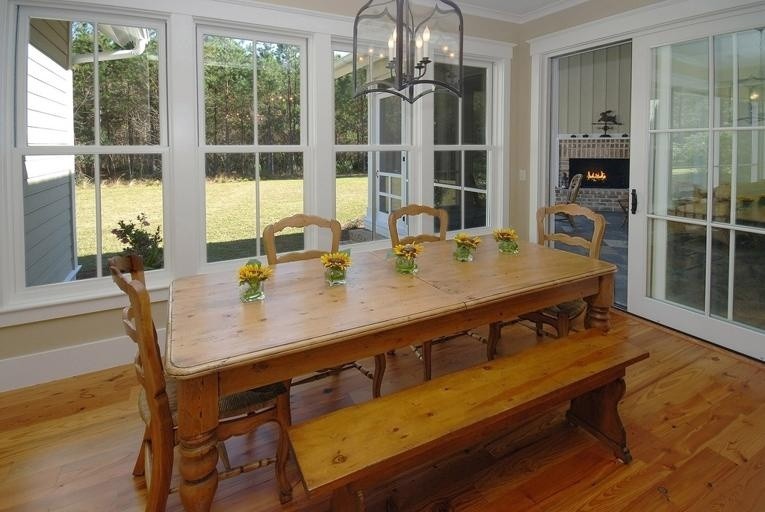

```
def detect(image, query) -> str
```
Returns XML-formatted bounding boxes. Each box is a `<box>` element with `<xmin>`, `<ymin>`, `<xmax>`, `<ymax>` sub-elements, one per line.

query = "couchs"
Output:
<box><xmin>676</xmin><ymin>180</ymin><xmax>765</xmax><ymax>242</ymax></box>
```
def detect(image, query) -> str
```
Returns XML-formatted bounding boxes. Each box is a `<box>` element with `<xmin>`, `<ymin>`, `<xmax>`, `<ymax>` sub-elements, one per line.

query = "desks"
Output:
<box><xmin>164</xmin><ymin>233</ymin><xmax>618</xmax><ymax>512</ymax></box>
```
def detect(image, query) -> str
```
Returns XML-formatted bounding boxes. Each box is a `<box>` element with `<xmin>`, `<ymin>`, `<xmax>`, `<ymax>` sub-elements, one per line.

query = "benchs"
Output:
<box><xmin>282</xmin><ymin>327</ymin><xmax>650</xmax><ymax>512</ymax></box>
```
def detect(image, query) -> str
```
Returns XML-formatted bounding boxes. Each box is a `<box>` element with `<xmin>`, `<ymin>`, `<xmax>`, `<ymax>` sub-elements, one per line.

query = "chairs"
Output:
<box><xmin>387</xmin><ymin>205</ymin><xmax>494</xmax><ymax>383</ymax></box>
<box><xmin>619</xmin><ymin>198</ymin><xmax>631</xmax><ymax>228</ymax></box>
<box><xmin>556</xmin><ymin>174</ymin><xmax>583</xmax><ymax>229</ymax></box>
<box><xmin>488</xmin><ymin>202</ymin><xmax>606</xmax><ymax>361</ymax></box>
<box><xmin>107</xmin><ymin>254</ymin><xmax>291</xmax><ymax>512</ymax></box>
<box><xmin>262</xmin><ymin>214</ymin><xmax>388</xmax><ymax>401</ymax></box>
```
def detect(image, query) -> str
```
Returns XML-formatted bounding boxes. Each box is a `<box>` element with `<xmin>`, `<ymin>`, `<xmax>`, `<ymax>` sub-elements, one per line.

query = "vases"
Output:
<box><xmin>339</xmin><ymin>241</ymin><xmax>348</xmax><ymax>245</ymax></box>
<box><xmin>395</xmin><ymin>254</ymin><xmax>420</xmax><ymax>275</ymax></box>
<box><xmin>499</xmin><ymin>239</ymin><xmax>519</xmax><ymax>256</ymax></box>
<box><xmin>455</xmin><ymin>245</ymin><xmax>475</xmax><ymax>262</ymax></box>
<box><xmin>323</xmin><ymin>267</ymin><xmax>348</xmax><ymax>287</ymax></box>
<box><xmin>237</xmin><ymin>280</ymin><xmax>267</xmax><ymax>303</ymax></box>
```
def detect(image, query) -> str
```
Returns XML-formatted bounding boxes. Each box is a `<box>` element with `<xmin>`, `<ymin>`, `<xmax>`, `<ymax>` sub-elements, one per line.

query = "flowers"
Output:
<box><xmin>453</xmin><ymin>234</ymin><xmax>481</xmax><ymax>248</ymax></box>
<box><xmin>493</xmin><ymin>227</ymin><xmax>520</xmax><ymax>241</ymax></box>
<box><xmin>317</xmin><ymin>250</ymin><xmax>353</xmax><ymax>270</ymax></box>
<box><xmin>392</xmin><ymin>241</ymin><xmax>424</xmax><ymax>260</ymax></box>
<box><xmin>235</xmin><ymin>258</ymin><xmax>273</xmax><ymax>286</ymax></box>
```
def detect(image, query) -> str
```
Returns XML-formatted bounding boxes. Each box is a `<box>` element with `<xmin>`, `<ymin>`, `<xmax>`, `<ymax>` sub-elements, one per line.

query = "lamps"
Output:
<box><xmin>351</xmin><ymin>1</ymin><xmax>465</xmax><ymax>104</ymax></box>
<box><xmin>727</xmin><ymin>74</ymin><xmax>765</xmax><ymax>126</ymax></box>
